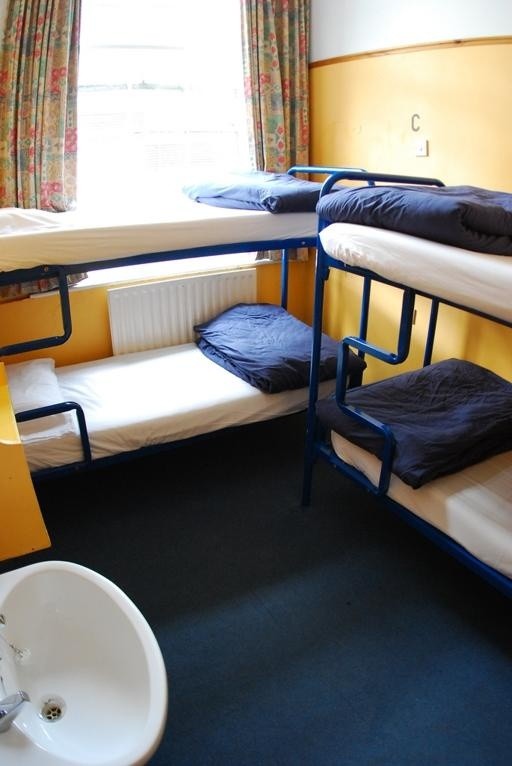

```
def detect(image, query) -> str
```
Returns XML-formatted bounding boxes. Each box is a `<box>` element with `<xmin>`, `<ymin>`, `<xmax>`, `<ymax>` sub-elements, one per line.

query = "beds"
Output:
<box><xmin>0</xmin><ymin>164</ymin><xmax>377</xmax><ymax>486</ymax></box>
<box><xmin>302</xmin><ymin>174</ymin><xmax>512</xmax><ymax>598</ymax></box>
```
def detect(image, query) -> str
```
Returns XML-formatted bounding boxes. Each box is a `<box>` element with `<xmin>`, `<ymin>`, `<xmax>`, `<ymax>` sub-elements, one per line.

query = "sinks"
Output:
<box><xmin>0</xmin><ymin>561</ymin><xmax>168</xmax><ymax>766</ymax></box>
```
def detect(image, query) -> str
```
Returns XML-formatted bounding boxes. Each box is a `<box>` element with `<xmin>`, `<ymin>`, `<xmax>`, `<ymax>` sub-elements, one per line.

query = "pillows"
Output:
<box><xmin>6</xmin><ymin>357</ymin><xmax>72</xmax><ymax>431</ymax></box>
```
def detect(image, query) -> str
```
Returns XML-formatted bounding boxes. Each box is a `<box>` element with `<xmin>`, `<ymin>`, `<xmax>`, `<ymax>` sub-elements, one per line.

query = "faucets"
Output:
<box><xmin>0</xmin><ymin>690</ymin><xmax>30</xmax><ymax>731</ymax></box>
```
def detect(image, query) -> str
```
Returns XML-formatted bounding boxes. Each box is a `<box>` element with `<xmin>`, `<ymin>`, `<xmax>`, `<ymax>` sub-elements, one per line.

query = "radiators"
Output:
<box><xmin>105</xmin><ymin>268</ymin><xmax>258</xmax><ymax>357</ymax></box>
